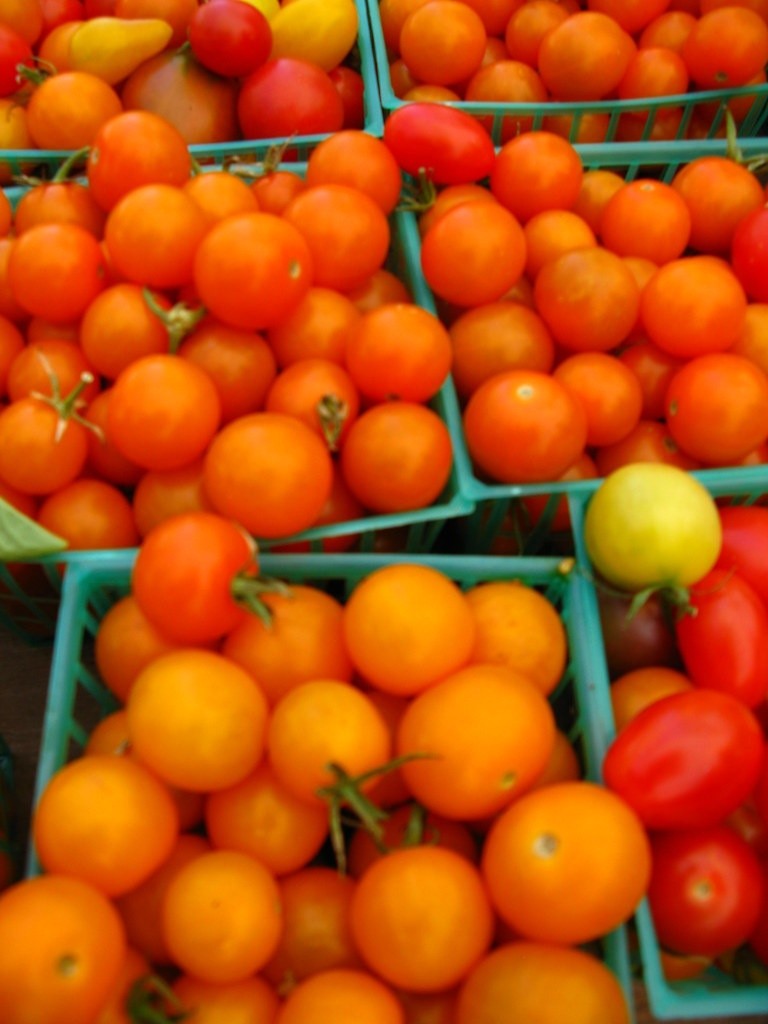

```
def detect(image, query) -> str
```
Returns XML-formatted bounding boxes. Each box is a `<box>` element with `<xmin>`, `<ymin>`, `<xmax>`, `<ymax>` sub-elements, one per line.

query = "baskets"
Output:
<box><xmin>31</xmin><ymin>547</ymin><xmax>636</xmax><ymax>1024</ymax></box>
<box><xmin>559</xmin><ymin>467</ymin><xmax>767</xmax><ymax>1021</ymax></box>
<box><xmin>0</xmin><ymin>1</ymin><xmax>385</xmax><ymax>181</ymax></box>
<box><xmin>366</xmin><ymin>0</ymin><xmax>767</xmax><ymax>155</ymax></box>
<box><xmin>0</xmin><ymin>164</ymin><xmax>477</xmax><ymax>599</ymax></box>
<box><xmin>408</xmin><ymin>138</ymin><xmax>767</xmax><ymax>551</ymax></box>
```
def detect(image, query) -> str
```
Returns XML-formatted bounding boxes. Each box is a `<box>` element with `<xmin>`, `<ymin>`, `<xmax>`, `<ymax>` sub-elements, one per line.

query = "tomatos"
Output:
<box><xmin>0</xmin><ymin>0</ymin><xmax>768</xmax><ymax>1024</ymax></box>
